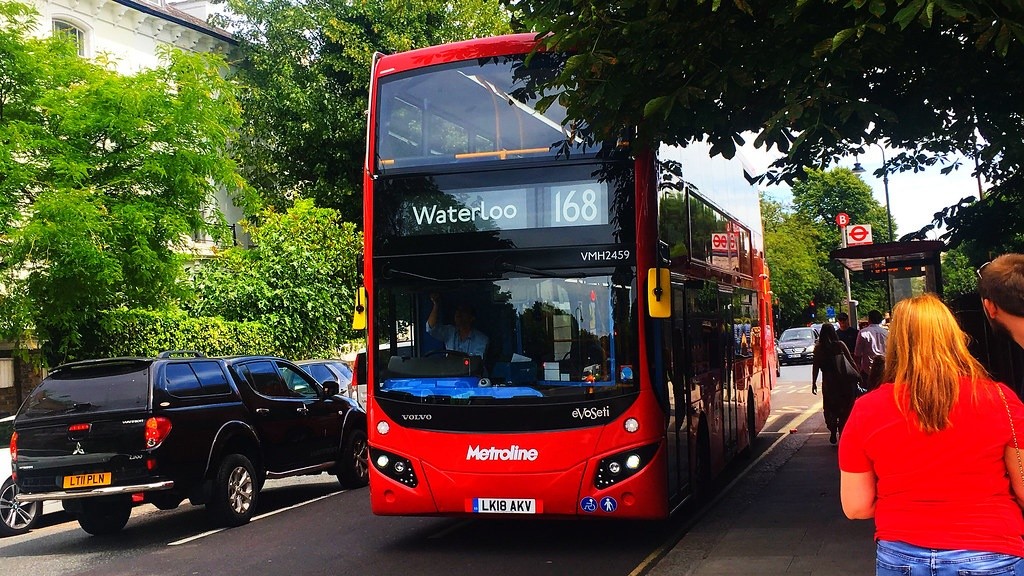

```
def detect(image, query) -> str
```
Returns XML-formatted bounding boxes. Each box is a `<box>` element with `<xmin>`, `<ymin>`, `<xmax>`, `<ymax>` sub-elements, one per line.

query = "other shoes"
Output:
<box><xmin>829</xmin><ymin>428</ymin><xmax>838</xmax><ymax>445</ymax></box>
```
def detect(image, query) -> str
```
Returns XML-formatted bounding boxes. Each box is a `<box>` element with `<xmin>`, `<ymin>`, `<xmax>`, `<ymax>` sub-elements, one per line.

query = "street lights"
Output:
<box><xmin>851</xmin><ymin>142</ymin><xmax>893</xmax><ymax>243</ymax></box>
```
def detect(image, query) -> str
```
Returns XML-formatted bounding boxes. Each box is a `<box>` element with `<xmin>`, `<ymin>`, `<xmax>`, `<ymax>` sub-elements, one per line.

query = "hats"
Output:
<box><xmin>837</xmin><ymin>312</ymin><xmax>848</xmax><ymax>321</ymax></box>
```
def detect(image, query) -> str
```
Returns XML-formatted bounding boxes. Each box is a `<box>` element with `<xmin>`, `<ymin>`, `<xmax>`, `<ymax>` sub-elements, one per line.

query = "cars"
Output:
<box><xmin>777</xmin><ymin>348</ymin><xmax>780</xmax><ymax>377</ymax></box>
<box><xmin>773</xmin><ymin>327</ymin><xmax>820</xmax><ymax>365</ymax></box>
<box><xmin>0</xmin><ymin>415</ymin><xmax>65</xmax><ymax>535</ymax></box>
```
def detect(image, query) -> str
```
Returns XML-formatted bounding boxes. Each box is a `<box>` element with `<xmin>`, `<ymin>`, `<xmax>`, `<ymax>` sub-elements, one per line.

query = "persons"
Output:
<box><xmin>854</xmin><ymin>309</ymin><xmax>889</xmax><ymax>387</ymax></box>
<box><xmin>425</xmin><ymin>293</ymin><xmax>490</xmax><ymax>379</ymax></box>
<box><xmin>734</xmin><ymin>318</ymin><xmax>752</xmax><ymax>354</ymax></box>
<box><xmin>838</xmin><ymin>291</ymin><xmax>1024</xmax><ymax>576</ymax></box>
<box><xmin>836</xmin><ymin>313</ymin><xmax>858</xmax><ymax>359</ymax></box>
<box><xmin>812</xmin><ymin>323</ymin><xmax>859</xmax><ymax>444</ymax></box>
<box><xmin>859</xmin><ymin>321</ymin><xmax>868</xmax><ymax>330</ymax></box>
<box><xmin>978</xmin><ymin>252</ymin><xmax>1023</xmax><ymax>349</ymax></box>
<box><xmin>881</xmin><ymin>310</ymin><xmax>891</xmax><ymax>325</ymax></box>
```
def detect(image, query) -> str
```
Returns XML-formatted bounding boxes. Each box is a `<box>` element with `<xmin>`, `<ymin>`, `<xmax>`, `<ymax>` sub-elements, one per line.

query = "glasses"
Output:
<box><xmin>977</xmin><ymin>262</ymin><xmax>991</xmax><ymax>281</ymax></box>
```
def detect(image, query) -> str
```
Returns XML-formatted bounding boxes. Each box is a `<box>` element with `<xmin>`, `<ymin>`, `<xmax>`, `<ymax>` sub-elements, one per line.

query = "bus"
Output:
<box><xmin>349</xmin><ymin>30</ymin><xmax>777</xmax><ymax>520</ymax></box>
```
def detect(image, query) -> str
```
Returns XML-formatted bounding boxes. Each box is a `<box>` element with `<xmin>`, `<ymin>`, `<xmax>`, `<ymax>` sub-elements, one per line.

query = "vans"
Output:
<box><xmin>351</xmin><ymin>342</ymin><xmax>417</xmax><ymax>412</ymax></box>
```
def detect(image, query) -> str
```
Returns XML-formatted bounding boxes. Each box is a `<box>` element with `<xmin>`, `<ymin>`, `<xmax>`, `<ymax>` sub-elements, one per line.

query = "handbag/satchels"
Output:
<box><xmin>834</xmin><ymin>349</ymin><xmax>862</xmax><ymax>384</ymax></box>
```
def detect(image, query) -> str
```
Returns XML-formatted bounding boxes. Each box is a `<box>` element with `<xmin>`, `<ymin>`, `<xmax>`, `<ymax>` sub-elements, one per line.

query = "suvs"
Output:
<box><xmin>810</xmin><ymin>322</ymin><xmax>841</xmax><ymax>334</ymax></box>
<box><xmin>10</xmin><ymin>351</ymin><xmax>367</xmax><ymax>536</ymax></box>
<box><xmin>280</xmin><ymin>359</ymin><xmax>353</xmax><ymax>397</ymax></box>
